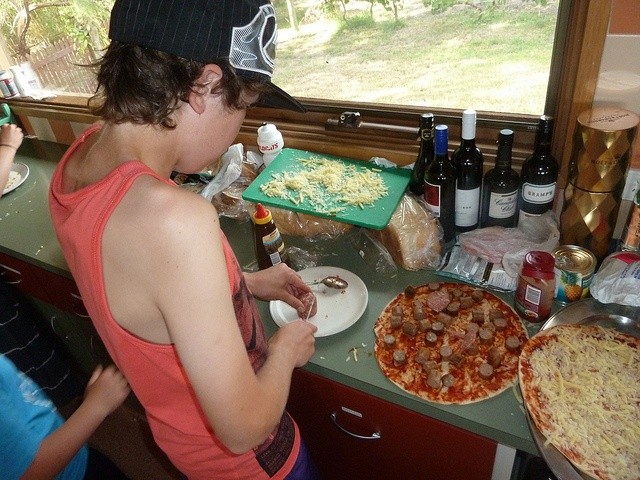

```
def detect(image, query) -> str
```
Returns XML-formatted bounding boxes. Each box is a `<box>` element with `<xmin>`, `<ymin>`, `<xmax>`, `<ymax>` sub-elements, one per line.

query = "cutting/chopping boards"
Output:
<box><xmin>241</xmin><ymin>147</ymin><xmax>415</xmax><ymax>232</ymax></box>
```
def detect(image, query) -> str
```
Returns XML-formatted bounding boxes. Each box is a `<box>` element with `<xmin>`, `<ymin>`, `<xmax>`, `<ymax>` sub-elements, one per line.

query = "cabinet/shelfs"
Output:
<box><xmin>0</xmin><ymin>251</ymin><xmax>30</xmax><ymax>305</ymax></box>
<box><xmin>283</xmin><ymin>370</ymin><xmax>516</xmax><ymax>480</ymax></box>
<box><xmin>32</xmin><ymin>267</ymin><xmax>151</xmax><ymax>422</ymax></box>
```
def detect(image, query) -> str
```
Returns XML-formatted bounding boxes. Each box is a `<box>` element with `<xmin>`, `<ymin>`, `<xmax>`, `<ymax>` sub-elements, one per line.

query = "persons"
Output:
<box><xmin>0</xmin><ymin>121</ymin><xmax>24</xmax><ymax>198</ymax></box>
<box><xmin>1</xmin><ymin>350</ymin><xmax>131</xmax><ymax>480</ymax></box>
<box><xmin>47</xmin><ymin>0</ymin><xmax>318</xmax><ymax>480</ymax></box>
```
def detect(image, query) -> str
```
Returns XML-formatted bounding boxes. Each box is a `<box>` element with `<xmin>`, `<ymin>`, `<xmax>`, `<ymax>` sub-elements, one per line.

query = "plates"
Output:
<box><xmin>268</xmin><ymin>266</ymin><xmax>369</xmax><ymax>342</ymax></box>
<box><xmin>0</xmin><ymin>157</ymin><xmax>29</xmax><ymax>198</ymax></box>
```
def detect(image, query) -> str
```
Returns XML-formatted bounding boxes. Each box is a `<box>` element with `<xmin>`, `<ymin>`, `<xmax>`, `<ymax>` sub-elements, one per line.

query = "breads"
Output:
<box><xmin>247</xmin><ymin>203</ymin><xmax>353</xmax><ymax>239</ymax></box>
<box><xmin>368</xmin><ymin>193</ymin><xmax>442</xmax><ymax>272</ymax></box>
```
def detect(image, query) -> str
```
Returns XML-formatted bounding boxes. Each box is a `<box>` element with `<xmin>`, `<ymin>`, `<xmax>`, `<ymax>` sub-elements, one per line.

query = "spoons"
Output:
<box><xmin>304</xmin><ymin>276</ymin><xmax>349</xmax><ymax>290</ymax></box>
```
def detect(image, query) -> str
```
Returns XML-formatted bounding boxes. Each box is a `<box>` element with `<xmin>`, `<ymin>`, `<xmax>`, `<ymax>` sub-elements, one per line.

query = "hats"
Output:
<box><xmin>108</xmin><ymin>0</ymin><xmax>306</xmax><ymax>114</ymax></box>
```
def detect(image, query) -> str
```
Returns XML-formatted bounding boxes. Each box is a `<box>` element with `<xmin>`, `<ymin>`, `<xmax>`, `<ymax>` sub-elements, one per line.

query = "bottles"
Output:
<box><xmin>514</xmin><ymin>250</ymin><xmax>557</xmax><ymax>324</ymax></box>
<box><xmin>516</xmin><ymin>114</ymin><xmax>559</xmax><ymax>227</ymax></box>
<box><xmin>10</xmin><ymin>65</ymin><xmax>31</xmax><ymax>98</ymax></box>
<box><xmin>20</xmin><ymin>61</ymin><xmax>44</xmax><ymax>95</ymax></box>
<box><xmin>251</xmin><ymin>202</ymin><xmax>292</xmax><ymax>275</ymax></box>
<box><xmin>480</xmin><ymin>128</ymin><xmax>521</xmax><ymax>229</ymax></box>
<box><xmin>452</xmin><ymin>108</ymin><xmax>484</xmax><ymax>233</ymax></box>
<box><xmin>406</xmin><ymin>112</ymin><xmax>436</xmax><ymax>205</ymax></box>
<box><xmin>423</xmin><ymin>123</ymin><xmax>457</xmax><ymax>245</ymax></box>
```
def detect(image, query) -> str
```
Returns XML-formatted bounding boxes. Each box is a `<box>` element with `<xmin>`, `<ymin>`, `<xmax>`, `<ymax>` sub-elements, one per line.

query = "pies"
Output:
<box><xmin>521</xmin><ymin>323</ymin><xmax>640</xmax><ymax>479</ymax></box>
<box><xmin>371</xmin><ymin>280</ymin><xmax>529</xmax><ymax>407</ymax></box>
